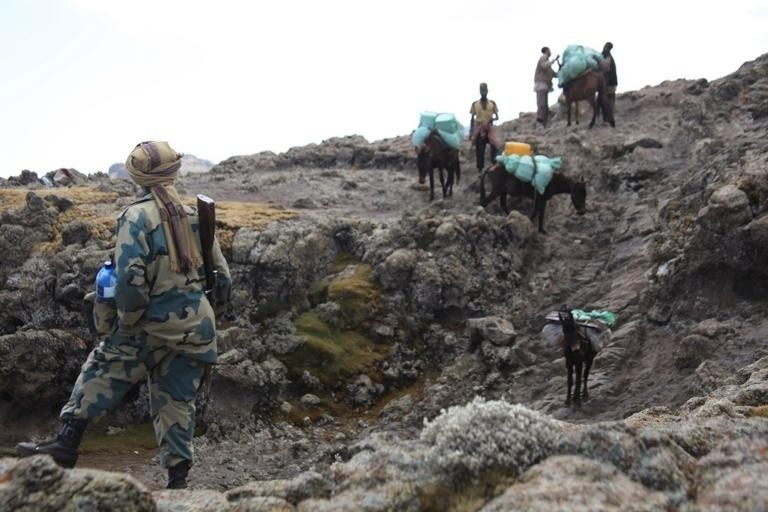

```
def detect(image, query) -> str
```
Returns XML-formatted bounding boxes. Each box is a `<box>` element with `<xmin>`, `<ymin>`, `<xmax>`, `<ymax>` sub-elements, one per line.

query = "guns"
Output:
<box><xmin>194</xmin><ymin>194</ymin><xmax>216</xmax><ymax>421</ymax></box>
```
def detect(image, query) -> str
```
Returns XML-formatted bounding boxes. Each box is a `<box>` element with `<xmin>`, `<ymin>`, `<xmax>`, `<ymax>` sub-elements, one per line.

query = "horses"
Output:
<box><xmin>557</xmin><ymin>309</ymin><xmax>595</xmax><ymax>407</ymax></box>
<box><xmin>476</xmin><ymin>160</ymin><xmax>587</xmax><ymax>234</ymax></box>
<box><xmin>414</xmin><ymin>130</ymin><xmax>462</xmax><ymax>203</ymax></box>
<box><xmin>562</xmin><ymin>69</ymin><xmax>607</xmax><ymax>128</ymax></box>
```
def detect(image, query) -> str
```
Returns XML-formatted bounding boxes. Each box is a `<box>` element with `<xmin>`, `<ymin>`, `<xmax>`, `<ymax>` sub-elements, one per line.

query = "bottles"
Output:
<box><xmin>94</xmin><ymin>262</ymin><xmax>118</xmax><ymax>301</ymax></box>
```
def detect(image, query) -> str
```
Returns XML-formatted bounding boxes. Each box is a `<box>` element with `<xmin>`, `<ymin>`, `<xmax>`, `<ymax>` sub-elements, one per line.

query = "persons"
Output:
<box><xmin>532</xmin><ymin>46</ymin><xmax>561</xmax><ymax>126</ymax></box>
<box><xmin>11</xmin><ymin>140</ymin><xmax>236</xmax><ymax>491</ymax></box>
<box><xmin>597</xmin><ymin>42</ymin><xmax>617</xmax><ymax>119</ymax></box>
<box><xmin>467</xmin><ymin>83</ymin><xmax>500</xmax><ymax>175</ymax></box>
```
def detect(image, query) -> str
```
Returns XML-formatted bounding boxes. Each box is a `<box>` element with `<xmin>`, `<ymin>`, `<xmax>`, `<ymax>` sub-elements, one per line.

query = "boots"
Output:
<box><xmin>15</xmin><ymin>417</ymin><xmax>87</xmax><ymax>470</ymax></box>
<box><xmin>166</xmin><ymin>461</ymin><xmax>188</xmax><ymax>490</ymax></box>
